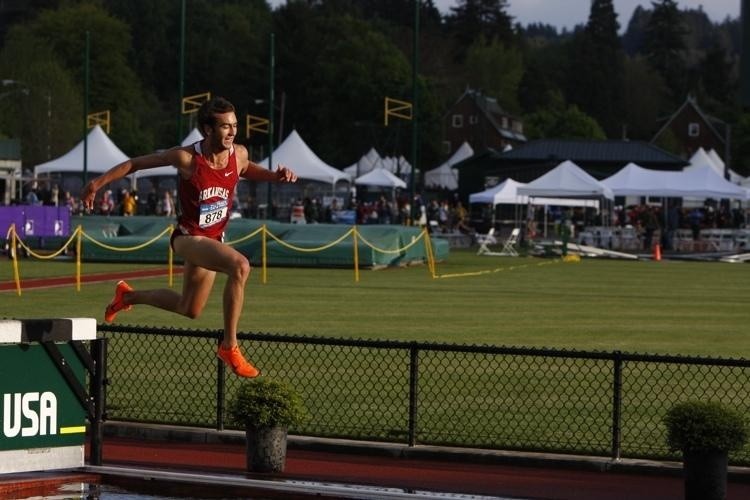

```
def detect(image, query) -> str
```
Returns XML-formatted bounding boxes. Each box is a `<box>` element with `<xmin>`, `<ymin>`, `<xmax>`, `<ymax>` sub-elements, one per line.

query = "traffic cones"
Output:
<box><xmin>653</xmin><ymin>243</ymin><xmax>661</xmax><ymax>261</ymax></box>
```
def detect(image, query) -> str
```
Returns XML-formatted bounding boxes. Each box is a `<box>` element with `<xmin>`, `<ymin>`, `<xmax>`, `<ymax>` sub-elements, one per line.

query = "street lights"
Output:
<box><xmin>1</xmin><ymin>76</ymin><xmax>54</xmax><ymax>193</ymax></box>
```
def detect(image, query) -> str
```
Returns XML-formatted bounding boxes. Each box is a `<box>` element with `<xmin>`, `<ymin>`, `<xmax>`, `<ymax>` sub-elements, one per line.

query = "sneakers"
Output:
<box><xmin>216</xmin><ymin>342</ymin><xmax>258</xmax><ymax>377</ymax></box>
<box><xmin>104</xmin><ymin>280</ymin><xmax>133</xmax><ymax>322</ymax></box>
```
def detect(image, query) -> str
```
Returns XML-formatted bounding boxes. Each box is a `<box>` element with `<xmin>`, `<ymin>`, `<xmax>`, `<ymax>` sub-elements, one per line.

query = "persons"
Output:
<box><xmin>81</xmin><ymin>96</ymin><xmax>297</xmax><ymax>378</ymax></box>
<box><xmin>523</xmin><ymin>204</ymin><xmax>750</xmax><ymax>251</ymax></box>
<box><xmin>25</xmin><ymin>181</ymin><xmax>178</xmax><ymax>216</ymax></box>
<box><xmin>291</xmin><ymin>194</ymin><xmax>500</xmax><ymax>235</ymax></box>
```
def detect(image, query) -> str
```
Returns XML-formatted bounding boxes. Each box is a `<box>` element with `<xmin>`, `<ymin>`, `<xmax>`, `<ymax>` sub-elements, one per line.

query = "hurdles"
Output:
<box><xmin>0</xmin><ymin>319</ymin><xmax>99</xmax><ymax>500</ymax></box>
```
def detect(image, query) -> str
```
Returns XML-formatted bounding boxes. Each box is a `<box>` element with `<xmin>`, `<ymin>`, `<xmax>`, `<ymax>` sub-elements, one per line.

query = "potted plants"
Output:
<box><xmin>226</xmin><ymin>376</ymin><xmax>313</xmax><ymax>473</ymax></box>
<box><xmin>662</xmin><ymin>400</ymin><xmax>747</xmax><ymax>500</ymax></box>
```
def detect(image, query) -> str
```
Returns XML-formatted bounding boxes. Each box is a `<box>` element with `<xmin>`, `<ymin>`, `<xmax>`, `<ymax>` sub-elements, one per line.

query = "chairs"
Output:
<box><xmin>501</xmin><ymin>228</ymin><xmax>520</xmax><ymax>256</ymax></box>
<box><xmin>578</xmin><ymin>226</ymin><xmax>750</xmax><ymax>252</ymax></box>
<box><xmin>475</xmin><ymin>226</ymin><xmax>496</xmax><ymax>255</ymax></box>
